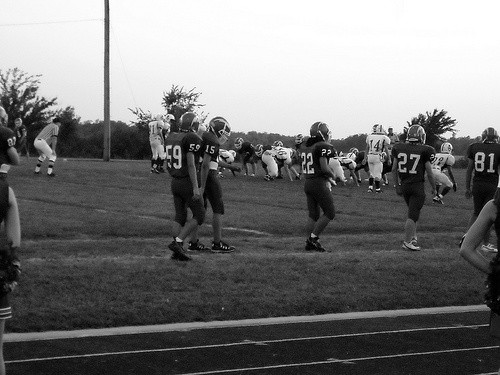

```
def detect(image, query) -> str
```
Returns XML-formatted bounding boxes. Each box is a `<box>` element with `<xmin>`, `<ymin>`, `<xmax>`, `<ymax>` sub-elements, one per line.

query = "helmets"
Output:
<box><xmin>273</xmin><ymin>140</ymin><xmax>284</xmax><ymax>147</ymax></box>
<box><xmin>234</xmin><ymin>137</ymin><xmax>244</xmax><ymax>149</ymax></box>
<box><xmin>255</xmin><ymin>144</ymin><xmax>263</xmax><ymax>152</ymax></box>
<box><xmin>209</xmin><ymin>117</ymin><xmax>231</xmax><ymax>139</ymax></box>
<box><xmin>310</xmin><ymin>122</ymin><xmax>329</xmax><ymax>141</ymax></box>
<box><xmin>179</xmin><ymin>112</ymin><xmax>200</xmax><ymax>132</ymax></box>
<box><xmin>407</xmin><ymin>125</ymin><xmax>426</xmax><ymax>145</ymax></box>
<box><xmin>481</xmin><ymin>127</ymin><xmax>500</xmax><ymax>143</ymax></box>
<box><xmin>441</xmin><ymin>142</ymin><xmax>453</xmax><ymax>153</ymax></box>
<box><xmin>155</xmin><ymin>114</ymin><xmax>175</xmax><ymax>123</ymax></box>
<box><xmin>372</xmin><ymin>124</ymin><xmax>385</xmax><ymax>135</ymax></box>
<box><xmin>0</xmin><ymin>106</ymin><xmax>8</xmax><ymax>123</ymax></box>
<box><xmin>295</xmin><ymin>134</ymin><xmax>305</xmax><ymax>144</ymax></box>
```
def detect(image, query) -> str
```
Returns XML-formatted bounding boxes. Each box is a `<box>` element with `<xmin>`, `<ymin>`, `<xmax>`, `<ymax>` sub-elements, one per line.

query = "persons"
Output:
<box><xmin>0</xmin><ymin>106</ymin><xmax>62</xmax><ymax>375</ymax></box>
<box><xmin>149</xmin><ymin>113</ymin><xmax>500</xmax><ymax>336</ymax></box>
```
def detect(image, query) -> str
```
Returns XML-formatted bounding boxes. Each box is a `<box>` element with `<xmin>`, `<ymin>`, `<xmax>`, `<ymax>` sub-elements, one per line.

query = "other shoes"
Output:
<box><xmin>481</xmin><ymin>243</ymin><xmax>498</xmax><ymax>253</ymax></box>
<box><xmin>305</xmin><ymin>232</ymin><xmax>326</xmax><ymax>252</ymax></box>
<box><xmin>188</xmin><ymin>239</ymin><xmax>236</xmax><ymax>253</ymax></box>
<box><xmin>459</xmin><ymin>234</ymin><xmax>467</xmax><ymax>248</ymax></box>
<box><xmin>34</xmin><ymin>170</ymin><xmax>43</xmax><ymax>176</ymax></box>
<box><xmin>151</xmin><ymin>167</ymin><xmax>165</xmax><ymax>174</ymax></box>
<box><xmin>401</xmin><ymin>235</ymin><xmax>421</xmax><ymax>251</ymax></box>
<box><xmin>47</xmin><ymin>172</ymin><xmax>55</xmax><ymax>176</ymax></box>
<box><xmin>329</xmin><ymin>173</ymin><xmax>401</xmax><ymax>194</ymax></box>
<box><xmin>218</xmin><ymin>168</ymin><xmax>301</xmax><ymax>182</ymax></box>
<box><xmin>168</xmin><ymin>236</ymin><xmax>192</xmax><ymax>261</ymax></box>
<box><xmin>432</xmin><ymin>195</ymin><xmax>445</xmax><ymax>205</ymax></box>
